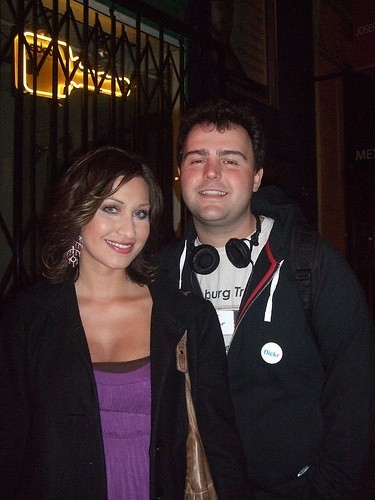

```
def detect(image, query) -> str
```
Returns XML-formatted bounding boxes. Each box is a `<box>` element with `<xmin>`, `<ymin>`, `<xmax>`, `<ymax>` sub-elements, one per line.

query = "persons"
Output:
<box><xmin>154</xmin><ymin>103</ymin><xmax>374</xmax><ymax>500</ymax></box>
<box><xmin>2</xmin><ymin>146</ymin><xmax>255</xmax><ymax>500</ymax></box>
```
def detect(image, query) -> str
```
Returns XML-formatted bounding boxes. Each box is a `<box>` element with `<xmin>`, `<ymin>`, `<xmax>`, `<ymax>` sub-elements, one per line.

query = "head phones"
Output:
<box><xmin>187</xmin><ymin>207</ymin><xmax>262</xmax><ymax>274</ymax></box>
